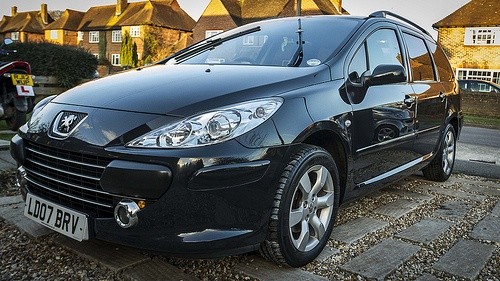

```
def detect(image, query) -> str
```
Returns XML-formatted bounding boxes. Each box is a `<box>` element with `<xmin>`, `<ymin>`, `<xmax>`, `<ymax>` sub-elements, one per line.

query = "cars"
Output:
<box><xmin>11</xmin><ymin>9</ymin><xmax>464</xmax><ymax>268</ymax></box>
<box><xmin>457</xmin><ymin>79</ymin><xmax>500</xmax><ymax>103</ymax></box>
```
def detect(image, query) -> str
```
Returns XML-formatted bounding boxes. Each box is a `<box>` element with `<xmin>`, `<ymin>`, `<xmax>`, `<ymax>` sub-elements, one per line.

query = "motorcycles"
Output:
<box><xmin>0</xmin><ymin>38</ymin><xmax>35</xmax><ymax>131</ymax></box>
